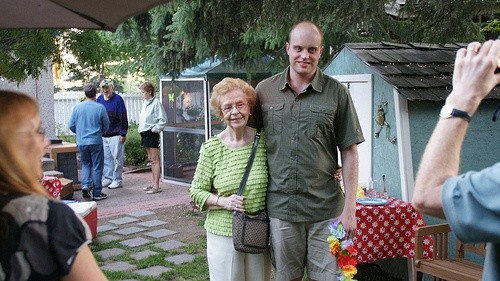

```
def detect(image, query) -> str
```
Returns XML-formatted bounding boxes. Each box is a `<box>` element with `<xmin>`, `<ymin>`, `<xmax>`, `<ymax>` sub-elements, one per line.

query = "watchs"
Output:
<box><xmin>439</xmin><ymin>106</ymin><xmax>471</xmax><ymax>122</ymax></box>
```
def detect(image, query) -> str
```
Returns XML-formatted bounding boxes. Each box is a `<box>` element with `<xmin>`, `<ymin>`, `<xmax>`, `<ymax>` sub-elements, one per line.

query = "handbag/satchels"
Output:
<box><xmin>232</xmin><ymin>211</ymin><xmax>270</xmax><ymax>254</ymax></box>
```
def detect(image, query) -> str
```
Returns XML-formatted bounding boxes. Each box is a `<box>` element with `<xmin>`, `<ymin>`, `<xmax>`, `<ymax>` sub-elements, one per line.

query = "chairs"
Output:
<box><xmin>413</xmin><ymin>223</ymin><xmax>486</xmax><ymax>281</ymax></box>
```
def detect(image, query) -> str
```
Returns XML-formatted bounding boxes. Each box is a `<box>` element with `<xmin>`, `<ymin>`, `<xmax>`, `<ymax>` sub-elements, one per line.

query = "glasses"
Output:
<box><xmin>16</xmin><ymin>128</ymin><xmax>46</xmax><ymax>141</ymax></box>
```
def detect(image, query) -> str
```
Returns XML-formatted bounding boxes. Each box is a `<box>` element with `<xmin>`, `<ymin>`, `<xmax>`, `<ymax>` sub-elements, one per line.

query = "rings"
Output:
<box><xmin>339</xmin><ymin>175</ymin><xmax>341</xmax><ymax>178</ymax></box>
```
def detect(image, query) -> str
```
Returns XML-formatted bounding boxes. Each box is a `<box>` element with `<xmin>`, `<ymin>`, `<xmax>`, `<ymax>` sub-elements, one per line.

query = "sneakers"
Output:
<box><xmin>107</xmin><ymin>180</ymin><xmax>122</xmax><ymax>189</ymax></box>
<box><xmin>82</xmin><ymin>187</ymin><xmax>91</xmax><ymax>200</ymax></box>
<box><xmin>102</xmin><ymin>178</ymin><xmax>112</xmax><ymax>187</ymax></box>
<box><xmin>93</xmin><ymin>193</ymin><xmax>107</xmax><ymax>200</ymax></box>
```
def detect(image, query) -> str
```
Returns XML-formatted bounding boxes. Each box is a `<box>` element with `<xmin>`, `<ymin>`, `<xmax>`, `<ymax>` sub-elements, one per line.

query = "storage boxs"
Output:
<box><xmin>61</xmin><ymin>200</ymin><xmax>97</xmax><ymax>240</ymax></box>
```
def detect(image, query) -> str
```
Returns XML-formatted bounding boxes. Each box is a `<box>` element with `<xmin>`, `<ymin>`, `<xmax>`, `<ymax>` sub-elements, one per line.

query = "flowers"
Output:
<box><xmin>326</xmin><ymin>218</ymin><xmax>358</xmax><ymax>281</ymax></box>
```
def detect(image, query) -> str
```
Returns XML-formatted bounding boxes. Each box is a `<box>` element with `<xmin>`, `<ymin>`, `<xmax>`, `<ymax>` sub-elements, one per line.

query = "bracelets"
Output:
<box><xmin>216</xmin><ymin>197</ymin><xmax>220</xmax><ymax>208</ymax></box>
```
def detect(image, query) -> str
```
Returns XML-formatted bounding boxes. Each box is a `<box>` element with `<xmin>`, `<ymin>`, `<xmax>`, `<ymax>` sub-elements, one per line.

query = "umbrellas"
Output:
<box><xmin>0</xmin><ymin>0</ymin><xmax>169</xmax><ymax>33</ymax></box>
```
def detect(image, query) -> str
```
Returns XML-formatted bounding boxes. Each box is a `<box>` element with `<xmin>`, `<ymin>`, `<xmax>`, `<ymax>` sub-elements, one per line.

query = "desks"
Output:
<box><xmin>46</xmin><ymin>142</ymin><xmax>82</xmax><ymax>190</ymax></box>
<box><xmin>352</xmin><ymin>189</ymin><xmax>439</xmax><ymax>263</ymax></box>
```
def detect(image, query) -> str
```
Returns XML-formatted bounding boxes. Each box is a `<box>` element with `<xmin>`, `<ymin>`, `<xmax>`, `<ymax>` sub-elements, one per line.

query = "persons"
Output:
<box><xmin>411</xmin><ymin>38</ymin><xmax>500</xmax><ymax>281</ymax></box>
<box><xmin>0</xmin><ymin>90</ymin><xmax>109</xmax><ymax>281</ymax></box>
<box><xmin>138</xmin><ymin>82</ymin><xmax>167</xmax><ymax>194</ymax></box>
<box><xmin>190</xmin><ymin>77</ymin><xmax>343</xmax><ymax>281</ymax></box>
<box><xmin>96</xmin><ymin>79</ymin><xmax>128</xmax><ymax>190</ymax></box>
<box><xmin>69</xmin><ymin>83</ymin><xmax>110</xmax><ymax>200</ymax></box>
<box><xmin>246</xmin><ymin>21</ymin><xmax>365</xmax><ymax>281</ymax></box>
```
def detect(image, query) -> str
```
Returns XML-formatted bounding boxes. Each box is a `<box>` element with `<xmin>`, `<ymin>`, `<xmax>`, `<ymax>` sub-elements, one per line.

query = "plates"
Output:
<box><xmin>356</xmin><ymin>198</ymin><xmax>389</xmax><ymax>205</ymax></box>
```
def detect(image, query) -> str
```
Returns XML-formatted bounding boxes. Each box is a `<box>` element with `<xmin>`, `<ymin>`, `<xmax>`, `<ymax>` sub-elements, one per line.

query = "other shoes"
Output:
<box><xmin>144</xmin><ymin>185</ymin><xmax>153</xmax><ymax>191</ymax></box>
<box><xmin>147</xmin><ymin>187</ymin><xmax>162</xmax><ymax>194</ymax></box>
